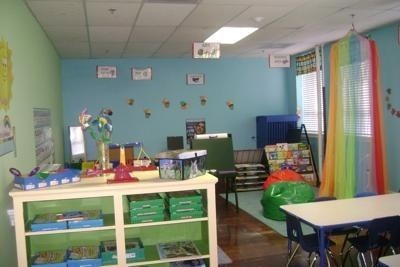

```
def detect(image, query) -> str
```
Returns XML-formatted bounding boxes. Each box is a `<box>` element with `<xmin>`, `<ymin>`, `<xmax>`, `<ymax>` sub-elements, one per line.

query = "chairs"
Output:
<box><xmin>279</xmin><ymin>190</ymin><xmax>400</xmax><ymax>267</ymax></box>
<box><xmin>191</xmin><ymin>135</ymin><xmax>238</xmax><ymax>212</ymax></box>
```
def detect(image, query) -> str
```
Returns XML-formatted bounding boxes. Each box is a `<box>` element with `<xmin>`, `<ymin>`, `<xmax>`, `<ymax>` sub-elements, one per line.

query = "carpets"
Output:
<box><xmin>218</xmin><ymin>190</ymin><xmax>318</xmax><ymax>237</ymax></box>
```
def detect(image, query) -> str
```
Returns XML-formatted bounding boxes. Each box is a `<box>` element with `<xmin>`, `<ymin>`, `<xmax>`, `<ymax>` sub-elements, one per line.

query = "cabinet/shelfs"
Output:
<box><xmin>9</xmin><ymin>169</ymin><xmax>218</xmax><ymax>267</ymax></box>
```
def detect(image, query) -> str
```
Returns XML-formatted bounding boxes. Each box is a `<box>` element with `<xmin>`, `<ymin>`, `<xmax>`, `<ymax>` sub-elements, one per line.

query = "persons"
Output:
<box><xmin>197</xmin><ymin>123</ymin><xmax>204</xmax><ymax>133</ymax></box>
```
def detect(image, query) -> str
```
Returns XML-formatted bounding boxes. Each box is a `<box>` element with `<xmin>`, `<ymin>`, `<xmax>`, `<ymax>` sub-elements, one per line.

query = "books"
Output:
<box><xmin>265</xmin><ymin>142</ymin><xmax>314</xmax><ymax>181</ymax></box>
